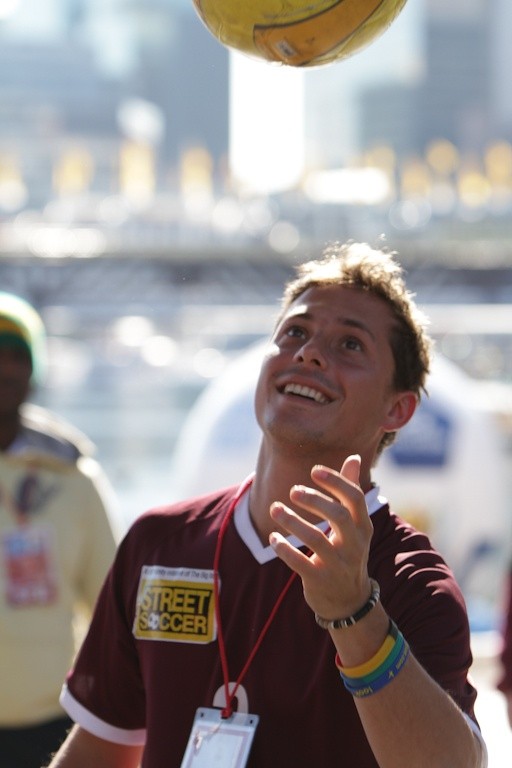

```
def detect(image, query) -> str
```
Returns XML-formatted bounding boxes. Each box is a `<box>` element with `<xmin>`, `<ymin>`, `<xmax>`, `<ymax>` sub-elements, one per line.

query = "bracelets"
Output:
<box><xmin>313</xmin><ymin>579</ymin><xmax>380</xmax><ymax>628</ymax></box>
<box><xmin>334</xmin><ymin>619</ymin><xmax>416</xmax><ymax>699</ymax></box>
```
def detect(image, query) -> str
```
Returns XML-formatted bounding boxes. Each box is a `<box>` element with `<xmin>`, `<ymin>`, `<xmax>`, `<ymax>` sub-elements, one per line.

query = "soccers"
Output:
<box><xmin>191</xmin><ymin>0</ymin><xmax>404</xmax><ymax>67</ymax></box>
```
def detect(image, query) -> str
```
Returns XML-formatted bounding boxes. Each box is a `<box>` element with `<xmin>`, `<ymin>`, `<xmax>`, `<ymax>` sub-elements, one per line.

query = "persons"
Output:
<box><xmin>0</xmin><ymin>290</ymin><xmax>119</xmax><ymax>768</ymax></box>
<box><xmin>43</xmin><ymin>240</ymin><xmax>490</xmax><ymax>768</ymax></box>
<box><xmin>496</xmin><ymin>567</ymin><xmax>512</xmax><ymax>730</ymax></box>
